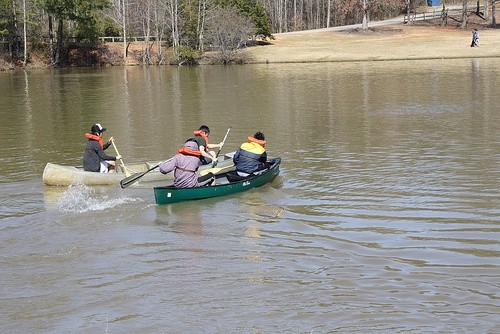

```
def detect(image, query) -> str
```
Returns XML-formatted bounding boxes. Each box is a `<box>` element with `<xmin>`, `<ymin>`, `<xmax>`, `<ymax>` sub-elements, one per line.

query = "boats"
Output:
<box><xmin>41</xmin><ymin>151</ymin><xmax>236</xmax><ymax>187</ymax></box>
<box><xmin>153</xmin><ymin>155</ymin><xmax>280</xmax><ymax>203</ymax></box>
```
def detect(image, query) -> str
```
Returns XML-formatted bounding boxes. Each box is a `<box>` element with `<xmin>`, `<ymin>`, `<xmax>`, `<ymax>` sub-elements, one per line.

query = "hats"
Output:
<box><xmin>91</xmin><ymin>123</ymin><xmax>107</xmax><ymax>133</ymax></box>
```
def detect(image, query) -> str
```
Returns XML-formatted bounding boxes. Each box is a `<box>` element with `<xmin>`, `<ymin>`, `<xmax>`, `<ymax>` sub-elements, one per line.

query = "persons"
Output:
<box><xmin>194</xmin><ymin>125</ymin><xmax>224</xmax><ymax>165</ymax></box>
<box><xmin>471</xmin><ymin>29</ymin><xmax>479</xmax><ymax>47</ymax></box>
<box><xmin>158</xmin><ymin>138</ymin><xmax>215</xmax><ymax>188</ymax></box>
<box><xmin>83</xmin><ymin>124</ymin><xmax>122</xmax><ymax>173</ymax></box>
<box><xmin>233</xmin><ymin>131</ymin><xmax>269</xmax><ymax>178</ymax></box>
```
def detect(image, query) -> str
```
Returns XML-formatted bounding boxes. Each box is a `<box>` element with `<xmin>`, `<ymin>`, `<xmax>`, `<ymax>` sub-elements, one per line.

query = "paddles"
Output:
<box><xmin>109</xmin><ymin>137</ymin><xmax>139</xmax><ymax>187</ymax></box>
<box><xmin>119</xmin><ymin>156</ymin><xmax>174</xmax><ymax>189</ymax></box>
<box><xmin>209</xmin><ymin>125</ymin><xmax>232</xmax><ymax>168</ymax></box>
<box><xmin>200</xmin><ymin>164</ymin><xmax>236</xmax><ymax>176</ymax></box>
